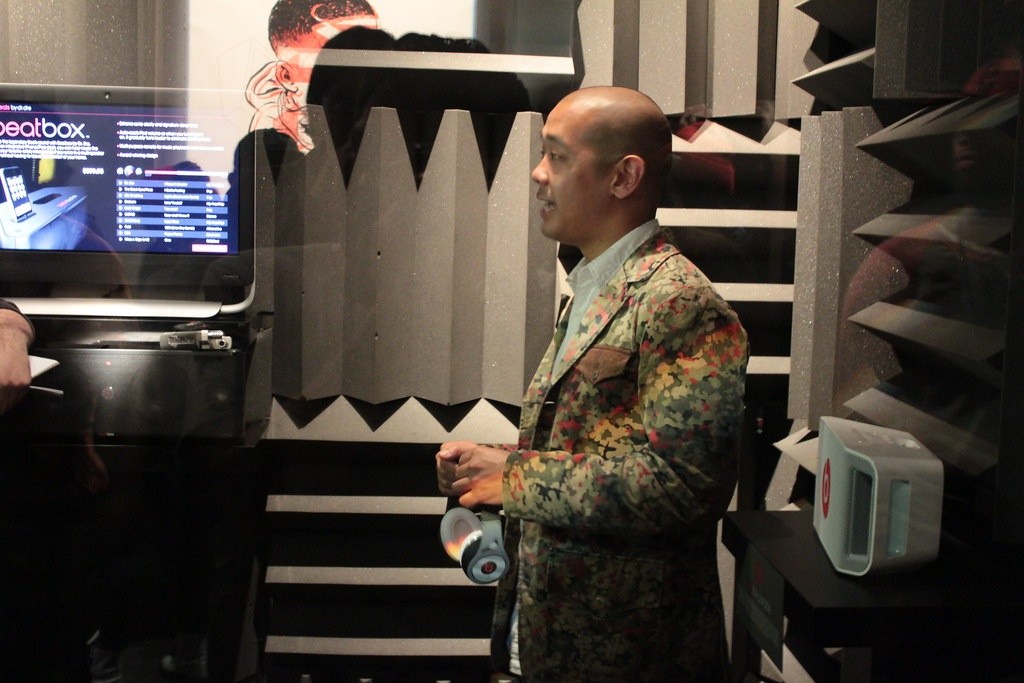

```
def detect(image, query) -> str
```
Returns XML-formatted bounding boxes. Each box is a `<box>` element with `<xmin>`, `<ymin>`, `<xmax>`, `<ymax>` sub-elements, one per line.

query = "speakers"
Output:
<box><xmin>812</xmin><ymin>415</ymin><xmax>943</xmax><ymax>577</ymax></box>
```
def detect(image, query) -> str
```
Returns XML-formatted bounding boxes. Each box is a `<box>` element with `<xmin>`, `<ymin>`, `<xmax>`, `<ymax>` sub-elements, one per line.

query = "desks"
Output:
<box><xmin>721</xmin><ymin>510</ymin><xmax>1024</xmax><ymax>683</ymax></box>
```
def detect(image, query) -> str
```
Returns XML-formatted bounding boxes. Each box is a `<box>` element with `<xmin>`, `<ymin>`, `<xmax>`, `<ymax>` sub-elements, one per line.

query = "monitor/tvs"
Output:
<box><xmin>1</xmin><ymin>81</ymin><xmax>256</xmax><ymax>348</ymax></box>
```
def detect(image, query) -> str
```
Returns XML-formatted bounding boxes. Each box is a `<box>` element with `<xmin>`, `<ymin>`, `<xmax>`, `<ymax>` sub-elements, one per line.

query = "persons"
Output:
<box><xmin>830</xmin><ymin>84</ymin><xmax>1024</xmax><ymax>437</ymax></box>
<box><xmin>435</xmin><ymin>85</ymin><xmax>748</xmax><ymax>682</ymax></box>
<box><xmin>1</xmin><ymin>126</ymin><xmax>300</xmax><ymax>682</ymax></box>
<box><xmin>0</xmin><ymin>298</ymin><xmax>36</xmax><ymax>414</ymax></box>
<box><xmin>657</xmin><ymin>101</ymin><xmax>800</xmax><ymax>284</ymax></box>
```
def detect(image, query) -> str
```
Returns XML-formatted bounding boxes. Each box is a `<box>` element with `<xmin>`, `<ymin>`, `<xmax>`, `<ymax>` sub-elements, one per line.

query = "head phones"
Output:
<box><xmin>439</xmin><ymin>494</ymin><xmax>510</xmax><ymax>584</ymax></box>
